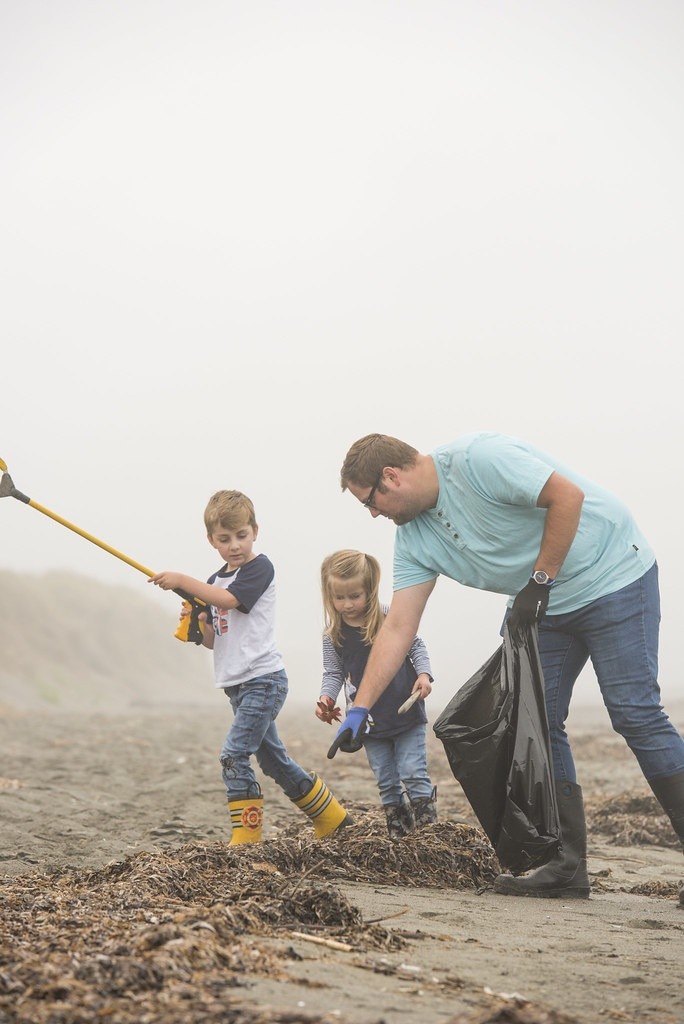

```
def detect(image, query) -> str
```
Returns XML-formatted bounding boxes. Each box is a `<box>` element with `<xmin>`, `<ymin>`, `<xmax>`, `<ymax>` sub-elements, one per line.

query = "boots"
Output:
<box><xmin>386</xmin><ymin>800</ymin><xmax>414</xmax><ymax>838</ymax></box>
<box><xmin>491</xmin><ymin>779</ymin><xmax>590</xmax><ymax>898</ymax></box>
<box><xmin>227</xmin><ymin>781</ymin><xmax>264</xmax><ymax>845</ymax></box>
<box><xmin>289</xmin><ymin>771</ymin><xmax>354</xmax><ymax>839</ymax></box>
<box><xmin>647</xmin><ymin>769</ymin><xmax>684</xmax><ymax>906</ymax></box>
<box><xmin>406</xmin><ymin>785</ymin><xmax>439</xmax><ymax>828</ymax></box>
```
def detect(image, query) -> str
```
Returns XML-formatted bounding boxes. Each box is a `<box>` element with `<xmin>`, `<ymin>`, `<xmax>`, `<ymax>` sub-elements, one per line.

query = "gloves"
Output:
<box><xmin>327</xmin><ymin>707</ymin><xmax>371</xmax><ymax>758</ymax></box>
<box><xmin>508</xmin><ymin>577</ymin><xmax>550</xmax><ymax>631</ymax></box>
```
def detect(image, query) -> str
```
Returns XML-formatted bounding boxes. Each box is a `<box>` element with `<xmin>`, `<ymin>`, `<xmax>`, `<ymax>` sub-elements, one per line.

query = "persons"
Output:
<box><xmin>315</xmin><ymin>549</ymin><xmax>439</xmax><ymax>838</ymax></box>
<box><xmin>327</xmin><ymin>434</ymin><xmax>684</xmax><ymax>907</ymax></box>
<box><xmin>147</xmin><ymin>490</ymin><xmax>354</xmax><ymax>846</ymax></box>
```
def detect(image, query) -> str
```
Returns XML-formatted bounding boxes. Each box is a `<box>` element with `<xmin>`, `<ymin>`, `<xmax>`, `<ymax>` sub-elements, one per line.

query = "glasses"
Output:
<box><xmin>364</xmin><ymin>474</ymin><xmax>382</xmax><ymax>510</ymax></box>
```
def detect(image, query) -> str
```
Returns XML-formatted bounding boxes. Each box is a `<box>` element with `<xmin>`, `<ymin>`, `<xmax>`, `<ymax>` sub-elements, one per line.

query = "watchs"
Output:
<box><xmin>531</xmin><ymin>570</ymin><xmax>555</xmax><ymax>585</ymax></box>
